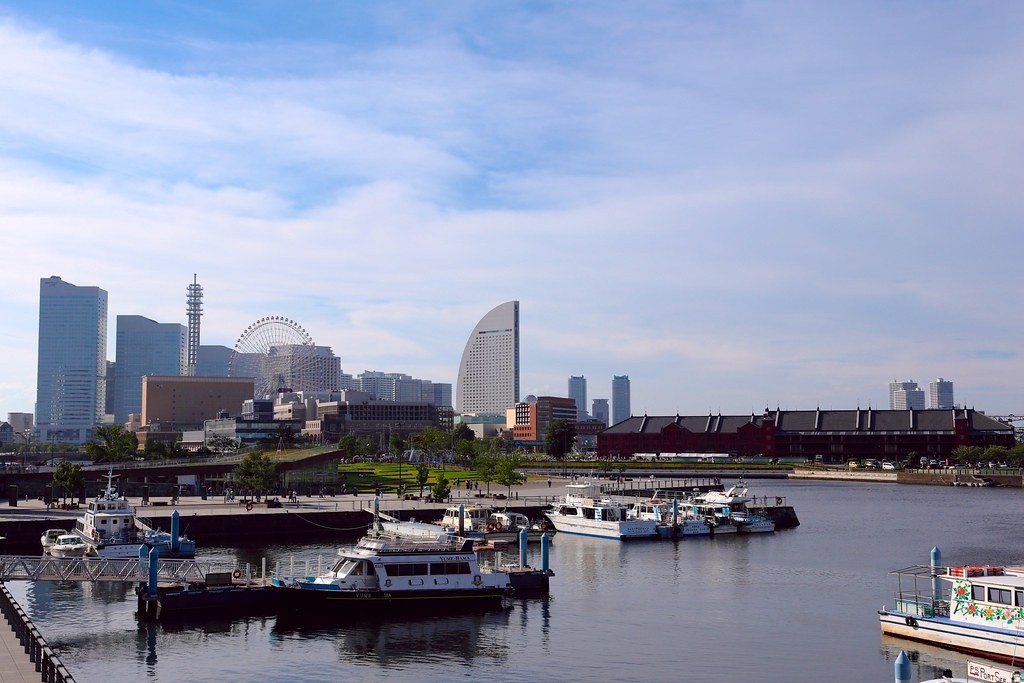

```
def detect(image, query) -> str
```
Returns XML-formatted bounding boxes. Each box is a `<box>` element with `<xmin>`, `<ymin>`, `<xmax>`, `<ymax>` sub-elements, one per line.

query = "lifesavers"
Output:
<box><xmin>777</xmin><ymin>497</ymin><xmax>783</xmax><ymax>504</ymax></box>
<box><xmin>540</xmin><ymin>521</ymin><xmax>549</xmax><ymax>530</ymax></box>
<box><xmin>91</xmin><ymin>528</ymin><xmax>94</xmax><ymax>537</ymax></box>
<box><xmin>487</xmin><ymin>522</ymin><xmax>495</xmax><ymax>531</ymax></box>
<box><xmin>905</xmin><ymin>614</ymin><xmax>914</xmax><ymax>626</ymax></box>
<box><xmin>689</xmin><ymin>516</ymin><xmax>693</xmax><ymax>520</ymax></box>
<box><xmin>233</xmin><ymin>570</ymin><xmax>242</xmax><ymax>579</ymax></box>
<box><xmin>246</xmin><ymin>503</ymin><xmax>253</xmax><ymax>511</ymax></box>
<box><xmin>495</xmin><ymin>521</ymin><xmax>502</xmax><ymax>530</ymax></box>
<box><xmin>696</xmin><ymin>515</ymin><xmax>700</xmax><ymax>520</ymax></box>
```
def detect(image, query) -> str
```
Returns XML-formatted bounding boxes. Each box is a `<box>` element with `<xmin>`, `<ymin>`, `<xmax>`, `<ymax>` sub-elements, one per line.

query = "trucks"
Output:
<box><xmin>814</xmin><ymin>454</ymin><xmax>824</xmax><ymax>465</ymax></box>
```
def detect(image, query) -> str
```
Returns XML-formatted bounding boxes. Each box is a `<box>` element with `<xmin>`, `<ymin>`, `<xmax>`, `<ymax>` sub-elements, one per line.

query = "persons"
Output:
<box><xmin>548</xmin><ymin>477</ymin><xmax>552</xmax><ymax>488</ymax></box>
<box><xmin>288</xmin><ymin>488</ymin><xmax>297</xmax><ymax>503</ymax></box>
<box><xmin>226</xmin><ymin>488</ymin><xmax>235</xmax><ymax>502</ymax></box>
<box><xmin>452</xmin><ymin>477</ymin><xmax>479</xmax><ymax>490</ymax></box>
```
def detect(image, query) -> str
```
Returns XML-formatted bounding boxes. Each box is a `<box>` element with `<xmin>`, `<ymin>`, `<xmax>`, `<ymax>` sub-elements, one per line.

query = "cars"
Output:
<box><xmin>847</xmin><ymin>454</ymin><xmax>1011</xmax><ymax>471</ymax></box>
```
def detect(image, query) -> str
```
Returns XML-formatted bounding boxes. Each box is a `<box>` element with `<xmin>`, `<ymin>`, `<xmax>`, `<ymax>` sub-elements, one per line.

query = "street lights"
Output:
<box><xmin>15</xmin><ymin>430</ymin><xmax>27</xmax><ymax>466</ymax></box>
<box><xmin>52</xmin><ymin>430</ymin><xmax>62</xmax><ymax>465</ymax></box>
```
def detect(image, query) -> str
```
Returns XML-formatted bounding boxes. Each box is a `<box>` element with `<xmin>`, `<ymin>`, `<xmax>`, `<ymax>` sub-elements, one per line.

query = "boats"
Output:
<box><xmin>698</xmin><ymin>504</ymin><xmax>740</xmax><ymax>535</ymax></box>
<box><xmin>54</xmin><ymin>536</ymin><xmax>85</xmax><ymax>558</ymax></box>
<box><xmin>38</xmin><ymin>530</ymin><xmax>67</xmax><ymax>550</ymax></box>
<box><xmin>894</xmin><ymin>650</ymin><xmax>1024</xmax><ymax>683</ymax></box>
<box><xmin>544</xmin><ymin>482</ymin><xmax>654</xmax><ymax>540</ymax></box>
<box><xmin>488</xmin><ymin>508</ymin><xmax>553</xmax><ymax>541</ymax></box>
<box><xmin>694</xmin><ymin>491</ymin><xmax>777</xmax><ymax>533</ymax></box>
<box><xmin>434</xmin><ymin>507</ymin><xmax>516</xmax><ymax>541</ymax></box>
<box><xmin>626</xmin><ymin>499</ymin><xmax>709</xmax><ymax>535</ymax></box>
<box><xmin>71</xmin><ymin>492</ymin><xmax>150</xmax><ymax>558</ymax></box>
<box><xmin>652</xmin><ymin>503</ymin><xmax>708</xmax><ymax>535</ymax></box>
<box><xmin>878</xmin><ymin>632</ymin><xmax>1024</xmax><ymax>681</ymax></box>
<box><xmin>875</xmin><ymin>546</ymin><xmax>1024</xmax><ymax>664</ymax></box>
<box><xmin>269</xmin><ymin>530</ymin><xmax>512</xmax><ymax>618</ymax></box>
